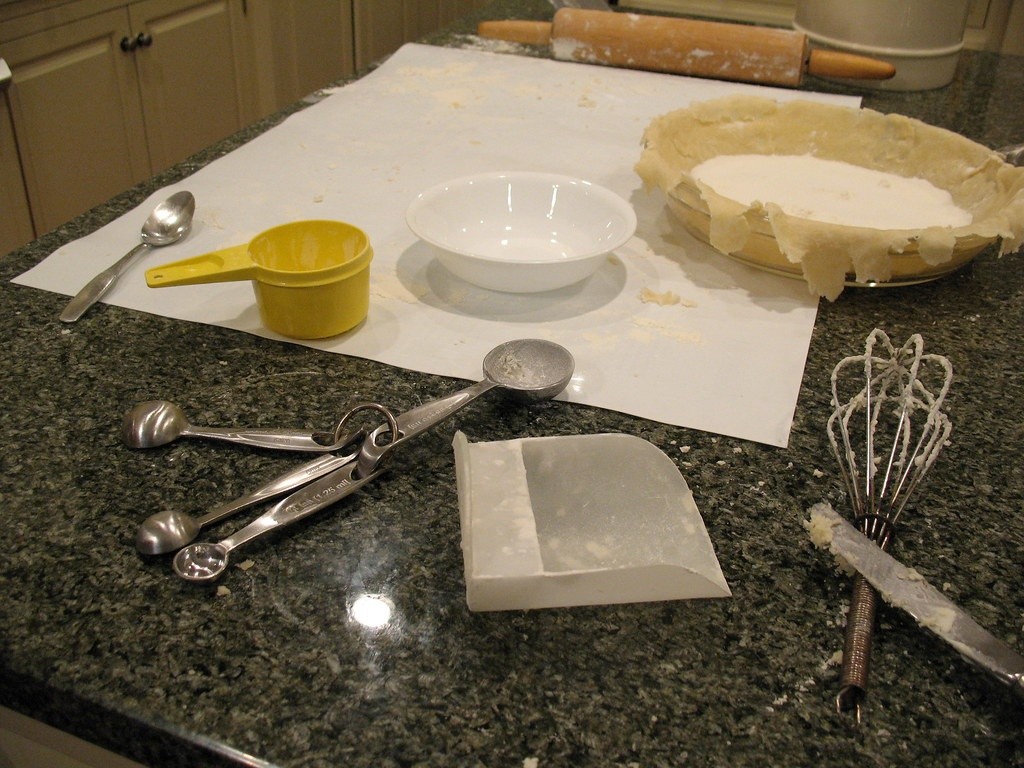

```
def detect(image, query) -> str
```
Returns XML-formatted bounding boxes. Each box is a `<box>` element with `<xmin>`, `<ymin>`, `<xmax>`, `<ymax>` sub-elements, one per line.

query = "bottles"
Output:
<box><xmin>795</xmin><ymin>0</ymin><xmax>968</xmax><ymax>92</ymax></box>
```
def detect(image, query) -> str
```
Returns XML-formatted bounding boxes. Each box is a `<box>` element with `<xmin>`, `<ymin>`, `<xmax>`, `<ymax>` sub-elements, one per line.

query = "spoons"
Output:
<box><xmin>135</xmin><ymin>430</ymin><xmax>372</xmax><ymax>555</ymax></box>
<box><xmin>120</xmin><ymin>401</ymin><xmax>348</xmax><ymax>450</ymax></box>
<box><xmin>174</xmin><ymin>460</ymin><xmax>391</xmax><ymax>580</ymax></box>
<box><xmin>60</xmin><ymin>190</ymin><xmax>195</xmax><ymax>323</ymax></box>
<box><xmin>358</xmin><ymin>339</ymin><xmax>575</xmax><ymax>478</ymax></box>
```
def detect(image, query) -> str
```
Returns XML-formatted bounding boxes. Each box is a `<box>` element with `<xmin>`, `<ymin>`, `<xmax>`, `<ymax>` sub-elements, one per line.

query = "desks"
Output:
<box><xmin>0</xmin><ymin>0</ymin><xmax>1024</xmax><ymax>766</ymax></box>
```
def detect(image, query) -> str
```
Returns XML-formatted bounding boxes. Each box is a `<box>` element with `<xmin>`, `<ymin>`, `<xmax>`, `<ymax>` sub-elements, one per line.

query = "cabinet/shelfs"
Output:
<box><xmin>0</xmin><ymin>0</ymin><xmax>497</xmax><ymax>261</ymax></box>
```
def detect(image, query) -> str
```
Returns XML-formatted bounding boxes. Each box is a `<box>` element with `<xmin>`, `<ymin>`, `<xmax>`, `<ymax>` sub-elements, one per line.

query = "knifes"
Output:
<box><xmin>808</xmin><ymin>503</ymin><xmax>1023</xmax><ymax>697</ymax></box>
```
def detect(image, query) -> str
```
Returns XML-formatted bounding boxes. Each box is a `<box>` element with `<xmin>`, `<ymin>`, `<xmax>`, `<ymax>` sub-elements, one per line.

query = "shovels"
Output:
<box><xmin>453</xmin><ymin>430</ymin><xmax>734</xmax><ymax>610</ymax></box>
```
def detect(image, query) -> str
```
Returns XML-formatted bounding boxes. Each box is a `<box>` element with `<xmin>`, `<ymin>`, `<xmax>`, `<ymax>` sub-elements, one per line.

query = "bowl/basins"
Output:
<box><xmin>406</xmin><ymin>171</ymin><xmax>637</xmax><ymax>293</ymax></box>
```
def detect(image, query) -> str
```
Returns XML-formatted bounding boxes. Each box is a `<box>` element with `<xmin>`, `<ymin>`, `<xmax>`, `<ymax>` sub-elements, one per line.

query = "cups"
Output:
<box><xmin>646</xmin><ymin>98</ymin><xmax>1008</xmax><ymax>287</ymax></box>
